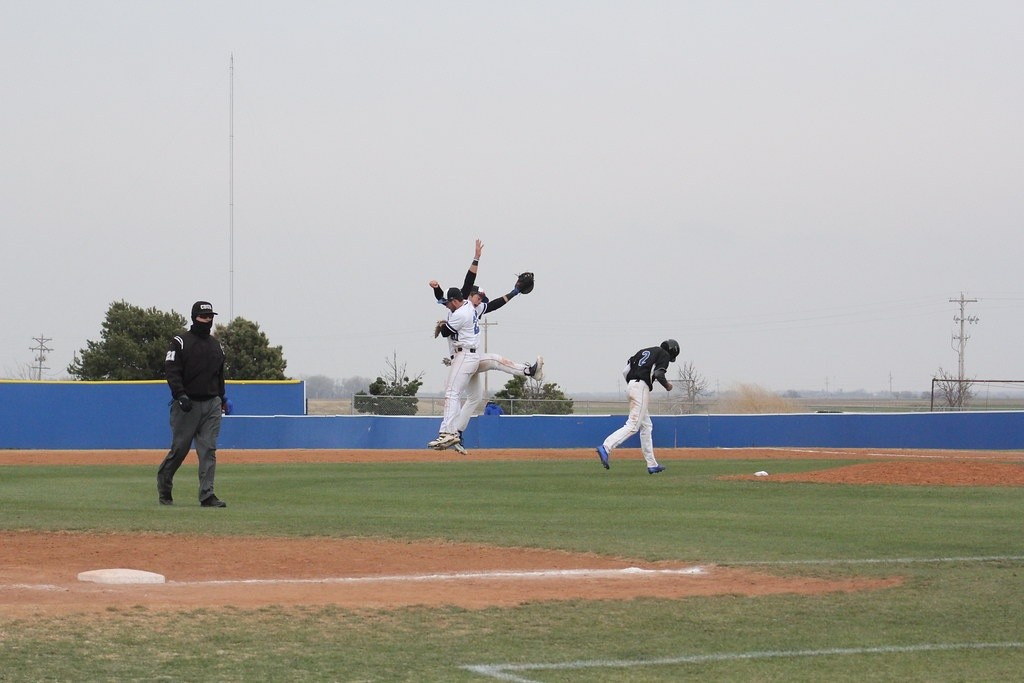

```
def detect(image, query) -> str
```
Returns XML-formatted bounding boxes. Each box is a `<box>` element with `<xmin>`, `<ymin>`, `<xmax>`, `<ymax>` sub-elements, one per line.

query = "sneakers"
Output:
<box><xmin>434</xmin><ymin>436</ymin><xmax>460</xmax><ymax>450</ymax></box>
<box><xmin>648</xmin><ymin>464</ymin><xmax>665</xmax><ymax>474</ymax></box>
<box><xmin>596</xmin><ymin>446</ymin><xmax>610</xmax><ymax>469</ymax></box>
<box><xmin>157</xmin><ymin>482</ymin><xmax>173</xmax><ymax>505</ymax></box>
<box><xmin>453</xmin><ymin>431</ymin><xmax>467</xmax><ymax>455</ymax></box>
<box><xmin>428</xmin><ymin>432</ymin><xmax>458</xmax><ymax>448</ymax></box>
<box><xmin>201</xmin><ymin>494</ymin><xmax>227</xmax><ymax>507</ymax></box>
<box><xmin>524</xmin><ymin>355</ymin><xmax>543</xmax><ymax>381</ymax></box>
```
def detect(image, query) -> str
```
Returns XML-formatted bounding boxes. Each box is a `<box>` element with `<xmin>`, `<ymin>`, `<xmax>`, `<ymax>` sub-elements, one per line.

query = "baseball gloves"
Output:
<box><xmin>514</xmin><ymin>272</ymin><xmax>534</xmax><ymax>294</ymax></box>
<box><xmin>435</xmin><ymin>320</ymin><xmax>447</xmax><ymax>339</ymax></box>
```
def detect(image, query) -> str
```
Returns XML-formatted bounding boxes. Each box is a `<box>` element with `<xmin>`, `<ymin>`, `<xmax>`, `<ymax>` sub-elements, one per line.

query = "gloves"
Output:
<box><xmin>222</xmin><ymin>399</ymin><xmax>233</xmax><ymax>415</ymax></box>
<box><xmin>176</xmin><ymin>393</ymin><xmax>193</xmax><ymax>412</ymax></box>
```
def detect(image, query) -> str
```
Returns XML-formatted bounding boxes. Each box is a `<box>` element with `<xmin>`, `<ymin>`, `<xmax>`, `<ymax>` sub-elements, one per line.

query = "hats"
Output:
<box><xmin>471</xmin><ymin>286</ymin><xmax>489</xmax><ymax>303</ymax></box>
<box><xmin>192</xmin><ymin>301</ymin><xmax>218</xmax><ymax>317</ymax></box>
<box><xmin>442</xmin><ymin>288</ymin><xmax>461</xmax><ymax>303</ymax></box>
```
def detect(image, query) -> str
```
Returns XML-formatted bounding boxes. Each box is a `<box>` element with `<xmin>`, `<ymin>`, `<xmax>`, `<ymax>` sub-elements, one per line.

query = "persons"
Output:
<box><xmin>595</xmin><ymin>339</ymin><xmax>680</xmax><ymax>475</ymax></box>
<box><xmin>427</xmin><ymin>238</ymin><xmax>544</xmax><ymax>455</ymax></box>
<box><xmin>157</xmin><ymin>301</ymin><xmax>233</xmax><ymax>508</ymax></box>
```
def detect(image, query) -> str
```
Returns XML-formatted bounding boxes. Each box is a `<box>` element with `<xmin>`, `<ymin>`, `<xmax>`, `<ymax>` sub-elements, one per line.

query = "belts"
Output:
<box><xmin>451</xmin><ymin>355</ymin><xmax>454</xmax><ymax>360</ymax></box>
<box><xmin>455</xmin><ymin>347</ymin><xmax>475</xmax><ymax>353</ymax></box>
<box><xmin>628</xmin><ymin>379</ymin><xmax>640</xmax><ymax>382</ymax></box>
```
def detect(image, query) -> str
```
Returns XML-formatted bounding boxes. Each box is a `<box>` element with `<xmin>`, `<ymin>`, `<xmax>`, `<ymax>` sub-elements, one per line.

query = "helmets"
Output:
<box><xmin>661</xmin><ymin>339</ymin><xmax>680</xmax><ymax>362</ymax></box>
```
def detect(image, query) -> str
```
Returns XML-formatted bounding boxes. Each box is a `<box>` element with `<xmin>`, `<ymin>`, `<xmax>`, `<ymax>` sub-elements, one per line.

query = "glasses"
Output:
<box><xmin>197</xmin><ymin>314</ymin><xmax>214</xmax><ymax>319</ymax></box>
